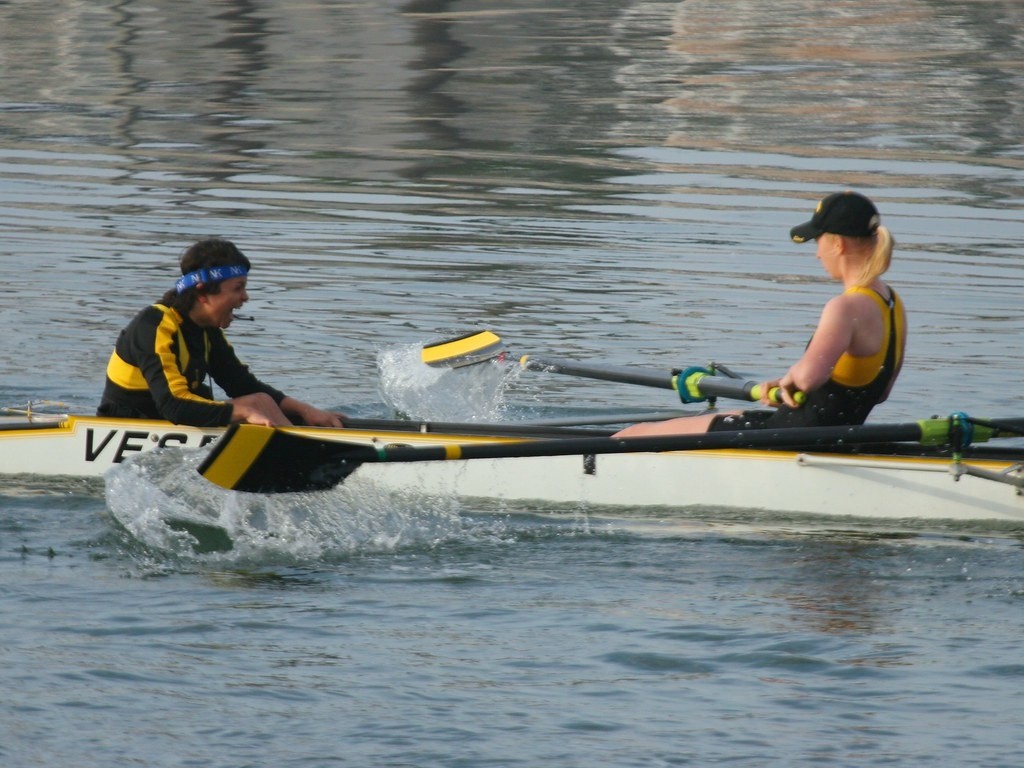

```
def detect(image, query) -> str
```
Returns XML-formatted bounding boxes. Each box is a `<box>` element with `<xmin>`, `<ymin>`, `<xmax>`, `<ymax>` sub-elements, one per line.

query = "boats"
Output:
<box><xmin>0</xmin><ymin>409</ymin><xmax>1024</xmax><ymax>524</ymax></box>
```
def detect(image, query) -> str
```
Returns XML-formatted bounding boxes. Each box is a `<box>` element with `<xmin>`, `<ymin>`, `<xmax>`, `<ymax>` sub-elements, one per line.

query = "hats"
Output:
<box><xmin>791</xmin><ymin>190</ymin><xmax>880</xmax><ymax>245</ymax></box>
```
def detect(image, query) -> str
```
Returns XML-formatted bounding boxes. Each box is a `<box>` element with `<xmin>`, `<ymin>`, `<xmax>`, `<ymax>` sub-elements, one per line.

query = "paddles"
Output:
<box><xmin>196</xmin><ymin>414</ymin><xmax>1024</xmax><ymax>494</ymax></box>
<box><xmin>421</xmin><ymin>329</ymin><xmax>805</xmax><ymax>406</ymax></box>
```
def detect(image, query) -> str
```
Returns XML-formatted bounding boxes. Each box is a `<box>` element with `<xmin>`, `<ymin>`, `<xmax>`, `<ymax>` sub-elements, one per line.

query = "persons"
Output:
<box><xmin>98</xmin><ymin>240</ymin><xmax>347</xmax><ymax>429</ymax></box>
<box><xmin>609</xmin><ymin>192</ymin><xmax>906</xmax><ymax>439</ymax></box>
